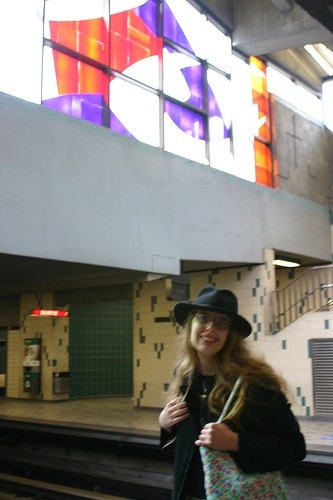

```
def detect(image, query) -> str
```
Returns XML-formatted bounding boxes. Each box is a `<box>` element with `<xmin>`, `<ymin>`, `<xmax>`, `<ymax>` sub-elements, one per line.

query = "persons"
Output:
<box><xmin>158</xmin><ymin>286</ymin><xmax>308</xmax><ymax>500</ymax></box>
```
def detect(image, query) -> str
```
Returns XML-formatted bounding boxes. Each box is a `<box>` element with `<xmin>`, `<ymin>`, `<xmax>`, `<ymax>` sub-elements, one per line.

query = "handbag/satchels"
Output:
<box><xmin>200</xmin><ymin>446</ymin><xmax>288</xmax><ymax>500</ymax></box>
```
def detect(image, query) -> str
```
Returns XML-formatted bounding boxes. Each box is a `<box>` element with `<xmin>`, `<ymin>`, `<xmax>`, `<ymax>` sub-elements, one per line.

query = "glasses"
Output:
<box><xmin>194</xmin><ymin>313</ymin><xmax>232</xmax><ymax>330</ymax></box>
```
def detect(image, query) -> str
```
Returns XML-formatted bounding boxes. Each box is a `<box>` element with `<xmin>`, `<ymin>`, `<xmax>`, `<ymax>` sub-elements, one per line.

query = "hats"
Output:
<box><xmin>173</xmin><ymin>286</ymin><xmax>253</xmax><ymax>342</ymax></box>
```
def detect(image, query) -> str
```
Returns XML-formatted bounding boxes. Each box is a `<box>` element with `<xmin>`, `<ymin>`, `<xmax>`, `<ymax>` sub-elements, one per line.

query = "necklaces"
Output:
<box><xmin>199</xmin><ymin>378</ymin><xmax>215</xmax><ymax>401</ymax></box>
